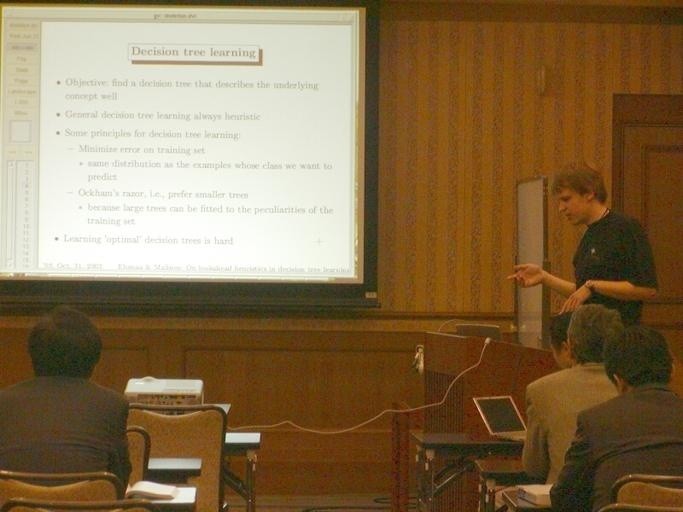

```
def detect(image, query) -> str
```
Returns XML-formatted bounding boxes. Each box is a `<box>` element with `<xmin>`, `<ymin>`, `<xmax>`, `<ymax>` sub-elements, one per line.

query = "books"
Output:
<box><xmin>124</xmin><ymin>480</ymin><xmax>177</xmax><ymax>501</ymax></box>
<box><xmin>514</xmin><ymin>484</ymin><xmax>554</xmax><ymax>504</ymax></box>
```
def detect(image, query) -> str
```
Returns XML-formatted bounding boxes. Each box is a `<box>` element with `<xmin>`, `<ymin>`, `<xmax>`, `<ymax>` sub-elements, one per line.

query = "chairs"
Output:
<box><xmin>594</xmin><ymin>501</ymin><xmax>683</xmax><ymax>512</ymax></box>
<box><xmin>600</xmin><ymin>474</ymin><xmax>683</xmax><ymax>507</ymax></box>
<box><xmin>0</xmin><ymin>403</ymin><xmax>228</xmax><ymax>512</ymax></box>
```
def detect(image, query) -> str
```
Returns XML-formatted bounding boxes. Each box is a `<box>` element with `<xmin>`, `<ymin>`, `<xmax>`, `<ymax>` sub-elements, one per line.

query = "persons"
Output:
<box><xmin>546</xmin><ymin>310</ymin><xmax>575</xmax><ymax>368</ymax></box>
<box><xmin>520</xmin><ymin>304</ymin><xmax>625</xmax><ymax>487</ymax></box>
<box><xmin>548</xmin><ymin>323</ymin><xmax>683</xmax><ymax>511</ymax></box>
<box><xmin>0</xmin><ymin>305</ymin><xmax>132</xmax><ymax>498</ymax></box>
<box><xmin>506</xmin><ymin>159</ymin><xmax>659</xmax><ymax>327</ymax></box>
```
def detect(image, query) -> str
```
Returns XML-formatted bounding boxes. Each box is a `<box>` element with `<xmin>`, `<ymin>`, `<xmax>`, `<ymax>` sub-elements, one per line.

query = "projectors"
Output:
<box><xmin>123</xmin><ymin>379</ymin><xmax>202</xmax><ymax>410</ymax></box>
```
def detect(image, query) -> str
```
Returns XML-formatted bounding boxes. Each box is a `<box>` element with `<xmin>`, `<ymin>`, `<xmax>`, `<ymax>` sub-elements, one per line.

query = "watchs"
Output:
<box><xmin>585</xmin><ymin>278</ymin><xmax>596</xmax><ymax>295</ymax></box>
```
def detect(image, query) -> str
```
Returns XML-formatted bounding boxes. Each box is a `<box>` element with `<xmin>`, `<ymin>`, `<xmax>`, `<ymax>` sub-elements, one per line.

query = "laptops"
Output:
<box><xmin>456</xmin><ymin>322</ymin><xmax>502</xmax><ymax>341</ymax></box>
<box><xmin>473</xmin><ymin>395</ymin><xmax>527</xmax><ymax>443</ymax></box>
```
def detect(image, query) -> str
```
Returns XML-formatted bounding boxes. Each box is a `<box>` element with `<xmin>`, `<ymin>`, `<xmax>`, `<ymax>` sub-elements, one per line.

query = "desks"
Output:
<box><xmin>410</xmin><ymin>432</ymin><xmax>550</xmax><ymax>512</ymax></box>
<box><xmin>223</xmin><ymin>432</ymin><xmax>261</xmax><ymax>512</ymax></box>
